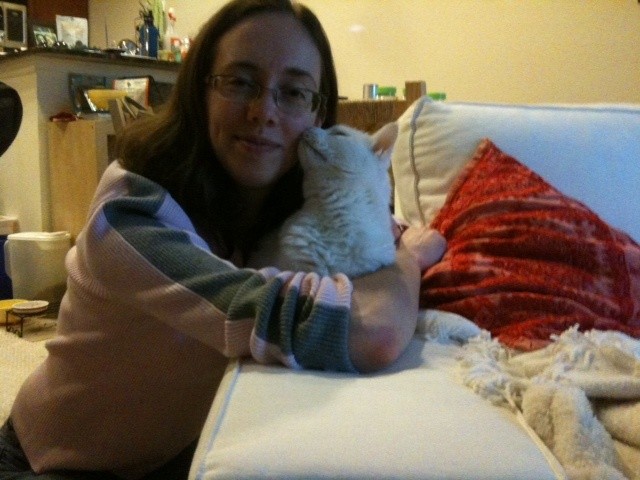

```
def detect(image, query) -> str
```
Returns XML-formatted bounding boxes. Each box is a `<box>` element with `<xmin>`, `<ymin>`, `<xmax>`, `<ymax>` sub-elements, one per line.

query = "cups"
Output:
<box><xmin>3</xmin><ymin>230</ymin><xmax>73</xmax><ymax>320</ymax></box>
<box><xmin>362</xmin><ymin>83</ymin><xmax>378</xmax><ymax>101</ymax></box>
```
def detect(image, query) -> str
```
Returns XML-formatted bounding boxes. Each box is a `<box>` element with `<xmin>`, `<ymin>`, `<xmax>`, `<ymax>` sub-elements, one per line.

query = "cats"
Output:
<box><xmin>270</xmin><ymin>121</ymin><xmax>485</xmax><ymax>347</ymax></box>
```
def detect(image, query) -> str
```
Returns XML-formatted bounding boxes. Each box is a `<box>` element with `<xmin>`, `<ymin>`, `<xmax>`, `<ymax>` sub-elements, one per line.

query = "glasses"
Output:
<box><xmin>204</xmin><ymin>72</ymin><xmax>326</xmax><ymax>117</ymax></box>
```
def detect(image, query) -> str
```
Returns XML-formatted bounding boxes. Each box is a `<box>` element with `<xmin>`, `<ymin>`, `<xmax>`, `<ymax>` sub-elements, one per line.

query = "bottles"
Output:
<box><xmin>427</xmin><ymin>92</ymin><xmax>448</xmax><ymax>103</ymax></box>
<box><xmin>137</xmin><ymin>14</ymin><xmax>158</xmax><ymax>59</ymax></box>
<box><xmin>376</xmin><ymin>86</ymin><xmax>399</xmax><ymax>101</ymax></box>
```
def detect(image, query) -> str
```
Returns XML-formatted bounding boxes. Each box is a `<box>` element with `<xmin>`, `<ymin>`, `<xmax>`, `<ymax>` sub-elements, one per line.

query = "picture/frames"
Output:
<box><xmin>0</xmin><ymin>0</ymin><xmax>29</xmax><ymax>50</ymax></box>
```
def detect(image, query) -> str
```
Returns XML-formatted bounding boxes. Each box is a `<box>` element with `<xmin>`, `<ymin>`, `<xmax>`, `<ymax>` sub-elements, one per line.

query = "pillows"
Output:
<box><xmin>392</xmin><ymin>94</ymin><xmax>640</xmax><ymax>253</ymax></box>
<box><xmin>410</xmin><ymin>139</ymin><xmax>640</xmax><ymax>354</ymax></box>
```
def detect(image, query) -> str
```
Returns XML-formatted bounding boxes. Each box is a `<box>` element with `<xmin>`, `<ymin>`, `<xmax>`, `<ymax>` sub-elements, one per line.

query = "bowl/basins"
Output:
<box><xmin>0</xmin><ymin>298</ymin><xmax>29</xmax><ymax>324</ymax></box>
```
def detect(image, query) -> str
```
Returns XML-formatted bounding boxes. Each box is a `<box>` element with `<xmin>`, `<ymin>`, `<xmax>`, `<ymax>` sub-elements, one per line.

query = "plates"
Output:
<box><xmin>11</xmin><ymin>299</ymin><xmax>49</xmax><ymax>319</ymax></box>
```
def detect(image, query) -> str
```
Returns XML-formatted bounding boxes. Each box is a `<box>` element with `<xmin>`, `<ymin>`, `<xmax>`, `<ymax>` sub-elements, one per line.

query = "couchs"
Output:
<box><xmin>190</xmin><ymin>169</ymin><xmax>637</xmax><ymax>480</ymax></box>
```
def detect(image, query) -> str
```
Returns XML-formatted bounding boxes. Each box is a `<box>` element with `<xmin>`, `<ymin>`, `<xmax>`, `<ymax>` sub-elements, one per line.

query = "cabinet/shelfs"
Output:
<box><xmin>48</xmin><ymin>110</ymin><xmax>142</xmax><ymax>249</ymax></box>
<box><xmin>1</xmin><ymin>47</ymin><xmax>183</xmax><ymax>233</ymax></box>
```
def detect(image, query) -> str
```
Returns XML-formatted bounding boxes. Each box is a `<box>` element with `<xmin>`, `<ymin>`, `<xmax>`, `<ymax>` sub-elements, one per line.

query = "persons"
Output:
<box><xmin>0</xmin><ymin>0</ymin><xmax>449</xmax><ymax>479</ymax></box>
